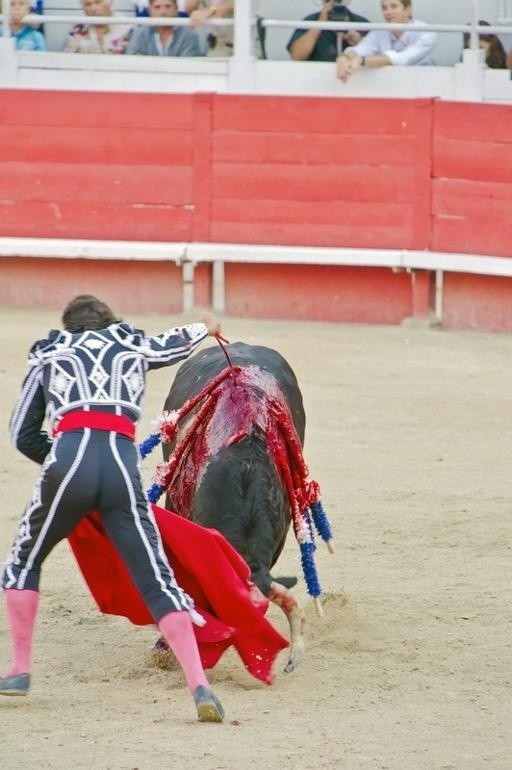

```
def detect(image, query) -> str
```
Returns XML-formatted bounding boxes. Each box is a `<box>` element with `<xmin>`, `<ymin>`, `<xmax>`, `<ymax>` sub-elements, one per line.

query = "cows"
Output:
<box><xmin>154</xmin><ymin>342</ymin><xmax>305</xmax><ymax>673</ymax></box>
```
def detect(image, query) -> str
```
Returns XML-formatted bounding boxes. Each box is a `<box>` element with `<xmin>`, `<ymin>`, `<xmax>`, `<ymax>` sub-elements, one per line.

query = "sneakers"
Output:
<box><xmin>0</xmin><ymin>670</ymin><xmax>33</xmax><ymax>697</ymax></box>
<box><xmin>191</xmin><ymin>683</ymin><xmax>226</xmax><ymax>725</ymax></box>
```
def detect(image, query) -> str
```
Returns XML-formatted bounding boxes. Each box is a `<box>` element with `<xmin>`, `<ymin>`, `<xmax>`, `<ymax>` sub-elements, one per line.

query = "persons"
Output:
<box><xmin>0</xmin><ymin>0</ymin><xmax>48</xmax><ymax>51</ymax></box>
<box><xmin>334</xmin><ymin>0</ymin><xmax>440</xmax><ymax>83</ymax></box>
<box><xmin>286</xmin><ymin>0</ymin><xmax>372</xmax><ymax>62</ymax></box>
<box><xmin>0</xmin><ymin>293</ymin><xmax>227</xmax><ymax>723</ymax></box>
<box><xmin>186</xmin><ymin>1</ymin><xmax>262</xmax><ymax>58</ymax></box>
<box><xmin>122</xmin><ymin>0</ymin><xmax>201</xmax><ymax>56</ymax></box>
<box><xmin>60</xmin><ymin>0</ymin><xmax>132</xmax><ymax>53</ymax></box>
<box><xmin>459</xmin><ymin>20</ymin><xmax>506</xmax><ymax>68</ymax></box>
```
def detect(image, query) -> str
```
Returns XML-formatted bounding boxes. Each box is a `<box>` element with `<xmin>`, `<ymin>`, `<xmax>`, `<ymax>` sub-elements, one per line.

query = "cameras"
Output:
<box><xmin>327</xmin><ymin>4</ymin><xmax>349</xmax><ymax>22</ymax></box>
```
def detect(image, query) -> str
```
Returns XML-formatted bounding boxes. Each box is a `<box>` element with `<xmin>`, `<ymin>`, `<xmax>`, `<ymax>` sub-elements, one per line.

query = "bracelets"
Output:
<box><xmin>361</xmin><ymin>56</ymin><xmax>366</xmax><ymax>66</ymax></box>
